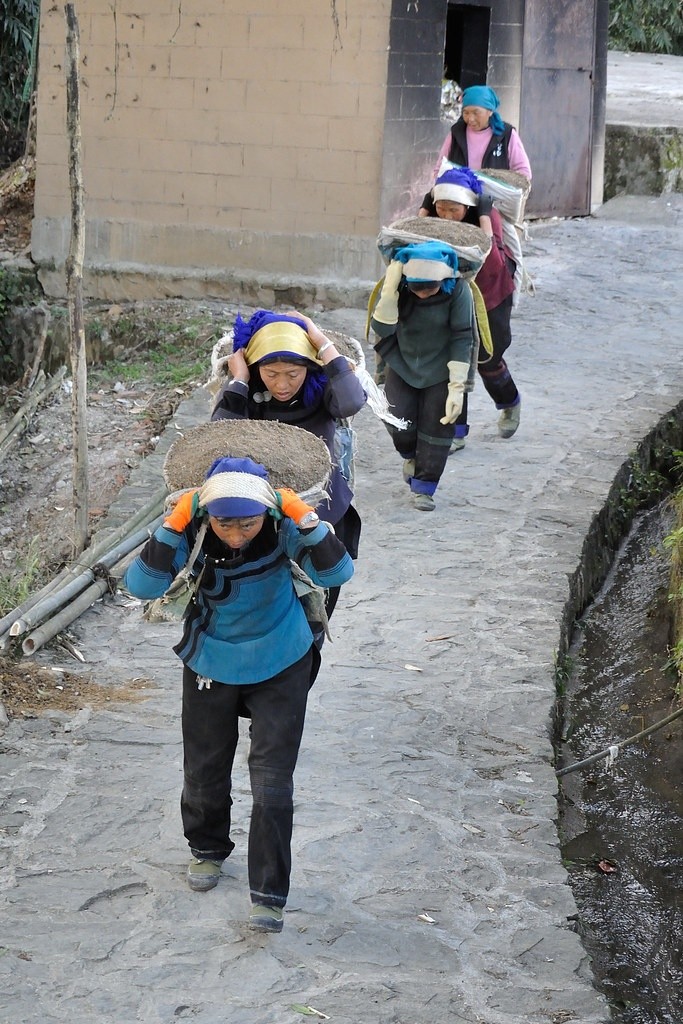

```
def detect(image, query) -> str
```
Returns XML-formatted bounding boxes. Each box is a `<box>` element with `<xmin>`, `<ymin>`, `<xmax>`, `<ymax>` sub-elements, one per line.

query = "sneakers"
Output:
<box><xmin>497</xmin><ymin>401</ymin><xmax>521</xmax><ymax>438</ymax></box>
<box><xmin>248</xmin><ymin>904</ymin><xmax>283</xmax><ymax>933</ymax></box>
<box><xmin>448</xmin><ymin>438</ymin><xmax>465</xmax><ymax>455</ymax></box>
<box><xmin>187</xmin><ymin>857</ymin><xmax>223</xmax><ymax>891</ymax></box>
<box><xmin>403</xmin><ymin>457</ymin><xmax>416</xmax><ymax>481</ymax></box>
<box><xmin>414</xmin><ymin>493</ymin><xmax>436</xmax><ymax>511</ymax></box>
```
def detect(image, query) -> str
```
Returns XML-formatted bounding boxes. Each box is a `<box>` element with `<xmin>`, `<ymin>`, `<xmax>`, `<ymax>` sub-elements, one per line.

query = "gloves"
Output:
<box><xmin>372</xmin><ymin>259</ymin><xmax>402</xmax><ymax>325</ymax></box>
<box><xmin>274</xmin><ymin>488</ymin><xmax>317</xmax><ymax>526</ymax></box>
<box><xmin>478</xmin><ymin>196</ymin><xmax>493</xmax><ymax>216</ymax></box>
<box><xmin>164</xmin><ymin>490</ymin><xmax>200</xmax><ymax>532</ymax></box>
<box><xmin>439</xmin><ymin>360</ymin><xmax>470</xmax><ymax>425</ymax></box>
<box><xmin>420</xmin><ymin>193</ymin><xmax>433</xmax><ymax>211</ymax></box>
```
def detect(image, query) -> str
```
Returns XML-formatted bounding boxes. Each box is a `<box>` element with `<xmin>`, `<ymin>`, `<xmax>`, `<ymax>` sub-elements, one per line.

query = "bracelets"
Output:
<box><xmin>317</xmin><ymin>342</ymin><xmax>335</xmax><ymax>359</ymax></box>
<box><xmin>229</xmin><ymin>380</ymin><xmax>249</xmax><ymax>391</ymax></box>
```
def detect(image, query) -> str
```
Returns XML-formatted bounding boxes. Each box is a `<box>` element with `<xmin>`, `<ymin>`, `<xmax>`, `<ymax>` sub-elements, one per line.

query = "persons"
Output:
<box><xmin>417</xmin><ymin>167</ymin><xmax>520</xmax><ymax>456</ymax></box>
<box><xmin>432</xmin><ymin>65</ymin><xmax>533</xmax><ymax>314</ymax></box>
<box><xmin>211</xmin><ymin>308</ymin><xmax>370</xmax><ymax>735</ymax></box>
<box><xmin>124</xmin><ymin>457</ymin><xmax>353</xmax><ymax>934</ymax></box>
<box><xmin>370</xmin><ymin>242</ymin><xmax>474</xmax><ymax>510</ymax></box>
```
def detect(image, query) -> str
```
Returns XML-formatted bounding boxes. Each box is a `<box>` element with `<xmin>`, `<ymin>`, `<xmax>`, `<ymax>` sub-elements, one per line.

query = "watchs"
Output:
<box><xmin>299</xmin><ymin>511</ymin><xmax>319</xmax><ymax>529</ymax></box>
